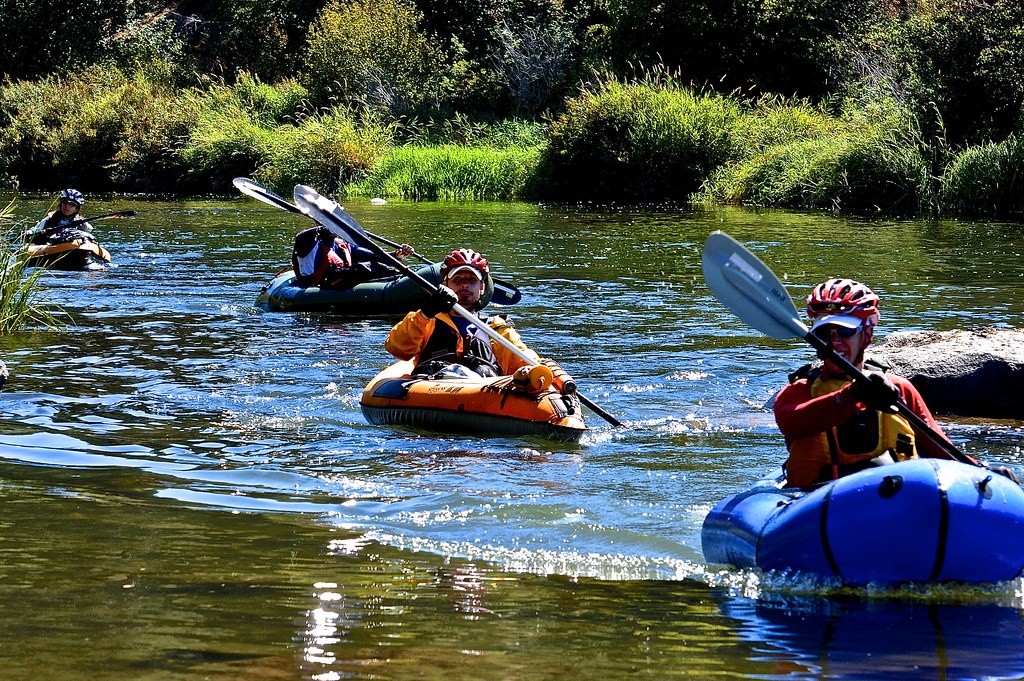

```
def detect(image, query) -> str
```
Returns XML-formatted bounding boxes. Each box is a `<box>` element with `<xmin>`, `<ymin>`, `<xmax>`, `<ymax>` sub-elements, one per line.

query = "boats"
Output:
<box><xmin>359</xmin><ymin>355</ymin><xmax>590</xmax><ymax>443</ymax></box>
<box><xmin>254</xmin><ymin>257</ymin><xmax>448</xmax><ymax>318</ymax></box>
<box><xmin>16</xmin><ymin>237</ymin><xmax>112</xmax><ymax>274</ymax></box>
<box><xmin>713</xmin><ymin>592</ymin><xmax>1024</xmax><ymax>681</ymax></box>
<box><xmin>699</xmin><ymin>461</ymin><xmax>1024</xmax><ymax>586</ymax></box>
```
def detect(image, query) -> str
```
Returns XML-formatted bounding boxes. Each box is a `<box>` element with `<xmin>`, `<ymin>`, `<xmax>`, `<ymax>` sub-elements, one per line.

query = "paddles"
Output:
<box><xmin>8</xmin><ymin>207</ymin><xmax>136</xmax><ymax>246</ymax></box>
<box><xmin>699</xmin><ymin>229</ymin><xmax>984</xmax><ymax>468</ymax></box>
<box><xmin>291</xmin><ymin>181</ymin><xmax>625</xmax><ymax>430</ymax></box>
<box><xmin>230</xmin><ymin>173</ymin><xmax>523</xmax><ymax>305</ymax></box>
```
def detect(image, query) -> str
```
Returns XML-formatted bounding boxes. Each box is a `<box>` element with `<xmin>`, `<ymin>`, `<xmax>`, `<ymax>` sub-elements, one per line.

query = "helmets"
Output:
<box><xmin>59</xmin><ymin>189</ymin><xmax>84</xmax><ymax>207</ymax></box>
<box><xmin>807</xmin><ymin>278</ymin><xmax>879</xmax><ymax>321</ymax></box>
<box><xmin>440</xmin><ymin>248</ymin><xmax>488</xmax><ymax>282</ymax></box>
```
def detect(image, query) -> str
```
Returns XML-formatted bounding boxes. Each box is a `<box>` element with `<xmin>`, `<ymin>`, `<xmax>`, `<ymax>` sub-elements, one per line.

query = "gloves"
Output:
<box><xmin>421</xmin><ymin>284</ymin><xmax>458</xmax><ymax>318</ymax></box>
<box><xmin>851</xmin><ymin>371</ymin><xmax>900</xmax><ymax>414</ymax></box>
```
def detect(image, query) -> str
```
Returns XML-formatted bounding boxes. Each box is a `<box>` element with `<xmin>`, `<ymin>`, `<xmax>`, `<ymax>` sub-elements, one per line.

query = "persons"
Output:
<box><xmin>292</xmin><ymin>200</ymin><xmax>415</xmax><ymax>286</ymax></box>
<box><xmin>774</xmin><ymin>278</ymin><xmax>1020</xmax><ymax>487</ymax></box>
<box><xmin>385</xmin><ymin>248</ymin><xmax>538</xmax><ymax>378</ymax></box>
<box><xmin>26</xmin><ymin>189</ymin><xmax>97</xmax><ymax>244</ymax></box>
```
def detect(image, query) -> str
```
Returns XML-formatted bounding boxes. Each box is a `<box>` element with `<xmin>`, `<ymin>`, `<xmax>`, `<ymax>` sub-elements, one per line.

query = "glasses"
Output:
<box><xmin>62</xmin><ymin>200</ymin><xmax>77</xmax><ymax>207</ymax></box>
<box><xmin>816</xmin><ymin>325</ymin><xmax>864</xmax><ymax>339</ymax></box>
